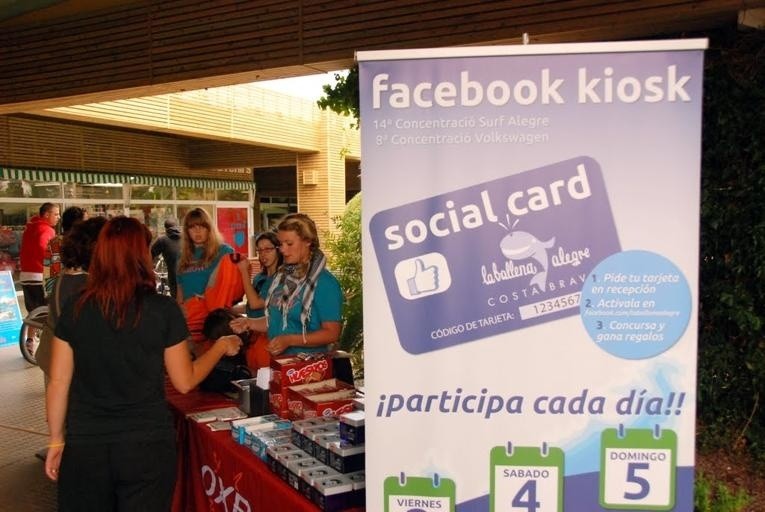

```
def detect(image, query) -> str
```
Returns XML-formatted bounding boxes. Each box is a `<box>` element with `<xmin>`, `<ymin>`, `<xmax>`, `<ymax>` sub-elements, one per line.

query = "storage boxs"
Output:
<box><xmin>267</xmin><ymin>355</ymin><xmax>357</xmax><ymax>421</ymax></box>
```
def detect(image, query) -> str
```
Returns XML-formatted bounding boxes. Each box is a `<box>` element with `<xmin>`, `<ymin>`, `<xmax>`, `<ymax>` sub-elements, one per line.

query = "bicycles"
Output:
<box><xmin>20</xmin><ymin>257</ymin><xmax>169</xmax><ymax>364</ymax></box>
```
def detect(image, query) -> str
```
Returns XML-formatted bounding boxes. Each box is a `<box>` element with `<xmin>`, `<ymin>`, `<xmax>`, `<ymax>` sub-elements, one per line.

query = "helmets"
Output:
<box><xmin>203</xmin><ymin>308</ymin><xmax>251</xmax><ymax>347</ymax></box>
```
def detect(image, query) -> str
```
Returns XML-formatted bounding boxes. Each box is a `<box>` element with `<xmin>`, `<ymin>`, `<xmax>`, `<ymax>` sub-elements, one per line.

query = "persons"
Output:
<box><xmin>42</xmin><ymin>214</ymin><xmax>244</xmax><ymax>512</ymax></box>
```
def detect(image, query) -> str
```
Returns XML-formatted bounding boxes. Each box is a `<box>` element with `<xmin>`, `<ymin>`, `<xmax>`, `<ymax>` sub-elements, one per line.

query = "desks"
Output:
<box><xmin>160</xmin><ymin>354</ymin><xmax>365</xmax><ymax>512</ymax></box>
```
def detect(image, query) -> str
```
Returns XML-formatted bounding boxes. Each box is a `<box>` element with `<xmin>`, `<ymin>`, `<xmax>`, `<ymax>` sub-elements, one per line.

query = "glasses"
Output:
<box><xmin>255</xmin><ymin>246</ymin><xmax>278</xmax><ymax>254</ymax></box>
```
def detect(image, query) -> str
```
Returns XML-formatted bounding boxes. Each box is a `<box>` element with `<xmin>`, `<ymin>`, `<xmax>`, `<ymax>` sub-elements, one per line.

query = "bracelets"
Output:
<box><xmin>46</xmin><ymin>442</ymin><xmax>65</xmax><ymax>447</ymax></box>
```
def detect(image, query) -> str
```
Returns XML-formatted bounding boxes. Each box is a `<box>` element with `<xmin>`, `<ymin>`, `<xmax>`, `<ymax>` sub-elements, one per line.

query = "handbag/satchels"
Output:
<box><xmin>199</xmin><ymin>358</ymin><xmax>253</xmax><ymax>393</ymax></box>
<box><xmin>35</xmin><ymin>313</ymin><xmax>56</xmax><ymax>374</ymax></box>
